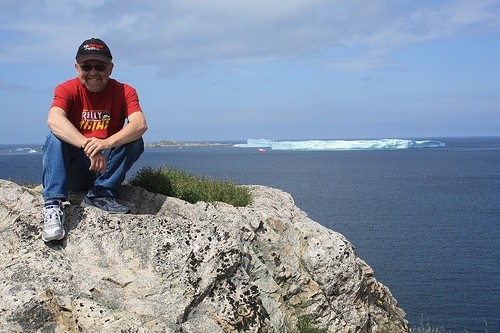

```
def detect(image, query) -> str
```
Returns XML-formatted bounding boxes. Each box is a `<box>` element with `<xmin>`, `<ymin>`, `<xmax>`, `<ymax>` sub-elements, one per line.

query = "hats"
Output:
<box><xmin>76</xmin><ymin>38</ymin><xmax>112</xmax><ymax>64</ymax></box>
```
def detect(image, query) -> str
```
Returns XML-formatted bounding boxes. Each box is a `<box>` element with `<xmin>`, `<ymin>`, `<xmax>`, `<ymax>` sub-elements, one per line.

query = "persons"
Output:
<box><xmin>40</xmin><ymin>38</ymin><xmax>148</xmax><ymax>243</ymax></box>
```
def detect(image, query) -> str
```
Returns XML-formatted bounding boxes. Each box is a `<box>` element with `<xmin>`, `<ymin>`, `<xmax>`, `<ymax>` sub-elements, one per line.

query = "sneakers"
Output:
<box><xmin>80</xmin><ymin>193</ymin><xmax>131</xmax><ymax>214</ymax></box>
<box><xmin>41</xmin><ymin>201</ymin><xmax>67</xmax><ymax>242</ymax></box>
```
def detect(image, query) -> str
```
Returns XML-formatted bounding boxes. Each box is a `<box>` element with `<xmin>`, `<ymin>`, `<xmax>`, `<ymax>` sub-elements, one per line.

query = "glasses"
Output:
<box><xmin>78</xmin><ymin>63</ymin><xmax>110</xmax><ymax>71</ymax></box>
